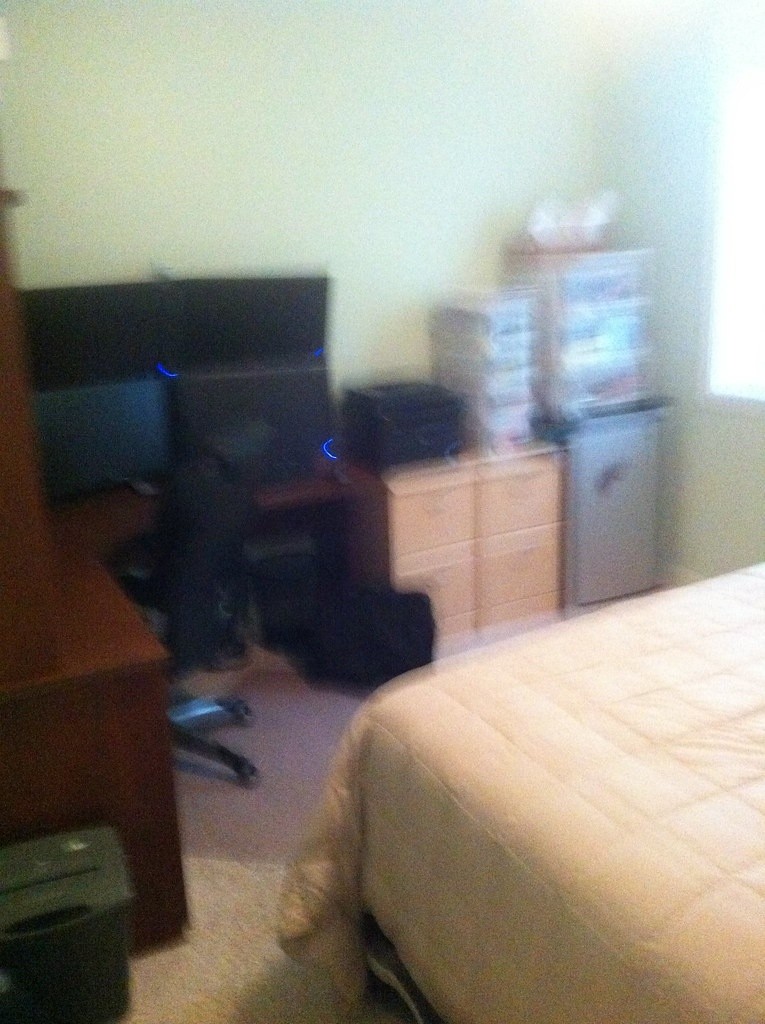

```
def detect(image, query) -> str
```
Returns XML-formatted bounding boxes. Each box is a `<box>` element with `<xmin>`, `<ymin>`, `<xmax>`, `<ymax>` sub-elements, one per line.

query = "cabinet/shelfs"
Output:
<box><xmin>0</xmin><ymin>179</ymin><xmax>189</xmax><ymax>944</ymax></box>
<box><xmin>341</xmin><ymin>445</ymin><xmax>565</xmax><ymax>659</ymax></box>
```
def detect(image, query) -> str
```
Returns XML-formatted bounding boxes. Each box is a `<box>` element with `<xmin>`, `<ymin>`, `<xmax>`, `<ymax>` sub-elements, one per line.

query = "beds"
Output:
<box><xmin>273</xmin><ymin>563</ymin><xmax>765</xmax><ymax>1023</ymax></box>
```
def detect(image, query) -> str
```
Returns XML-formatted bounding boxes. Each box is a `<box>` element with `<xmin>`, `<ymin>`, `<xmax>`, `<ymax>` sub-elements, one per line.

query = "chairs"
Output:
<box><xmin>96</xmin><ymin>430</ymin><xmax>262</xmax><ymax>790</ymax></box>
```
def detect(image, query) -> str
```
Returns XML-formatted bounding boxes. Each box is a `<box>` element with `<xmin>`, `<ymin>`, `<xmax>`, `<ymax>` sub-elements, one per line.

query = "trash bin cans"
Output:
<box><xmin>0</xmin><ymin>824</ymin><xmax>139</xmax><ymax>1024</ymax></box>
